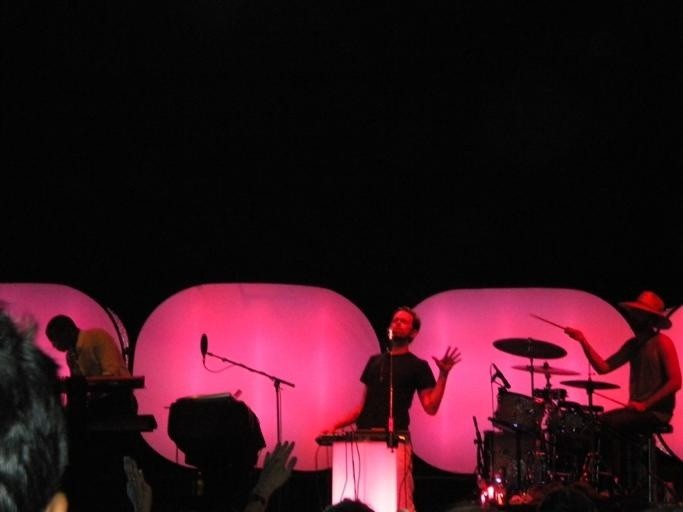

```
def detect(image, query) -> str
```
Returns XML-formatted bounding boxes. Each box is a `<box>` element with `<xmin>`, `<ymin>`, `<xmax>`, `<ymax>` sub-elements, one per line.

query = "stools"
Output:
<box><xmin>638</xmin><ymin>419</ymin><xmax>673</xmax><ymax>507</ymax></box>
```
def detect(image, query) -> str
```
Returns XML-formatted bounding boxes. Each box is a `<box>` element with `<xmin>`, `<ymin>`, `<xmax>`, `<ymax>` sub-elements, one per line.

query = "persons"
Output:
<box><xmin>320</xmin><ymin>306</ymin><xmax>462</xmax><ymax>436</ymax></box>
<box><xmin>44</xmin><ymin>315</ymin><xmax>135</xmax><ymax>381</ymax></box>
<box><xmin>243</xmin><ymin>438</ymin><xmax>299</xmax><ymax>512</ymax></box>
<box><xmin>0</xmin><ymin>309</ymin><xmax>72</xmax><ymax>512</ymax></box>
<box><xmin>563</xmin><ymin>291</ymin><xmax>683</xmax><ymax>442</ymax></box>
<box><xmin>121</xmin><ymin>455</ymin><xmax>154</xmax><ymax>512</ymax></box>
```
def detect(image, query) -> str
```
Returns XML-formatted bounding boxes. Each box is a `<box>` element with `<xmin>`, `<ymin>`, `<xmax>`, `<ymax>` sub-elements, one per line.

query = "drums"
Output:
<box><xmin>551</xmin><ymin>402</ymin><xmax>604</xmax><ymax>446</ymax></box>
<box><xmin>483</xmin><ymin>429</ymin><xmax>546</xmax><ymax>491</ymax></box>
<box><xmin>492</xmin><ymin>390</ymin><xmax>546</xmax><ymax>437</ymax></box>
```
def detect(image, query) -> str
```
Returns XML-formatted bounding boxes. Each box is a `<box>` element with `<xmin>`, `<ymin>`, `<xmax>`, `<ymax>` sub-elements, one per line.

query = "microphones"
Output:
<box><xmin>492</xmin><ymin>361</ymin><xmax>511</xmax><ymax>389</ymax></box>
<box><xmin>201</xmin><ymin>334</ymin><xmax>207</xmax><ymax>355</ymax></box>
<box><xmin>386</xmin><ymin>327</ymin><xmax>394</xmax><ymax>350</ymax></box>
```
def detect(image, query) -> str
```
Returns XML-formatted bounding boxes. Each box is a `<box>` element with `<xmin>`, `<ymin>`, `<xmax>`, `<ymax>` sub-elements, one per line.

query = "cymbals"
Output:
<box><xmin>512</xmin><ymin>365</ymin><xmax>579</xmax><ymax>376</ymax></box>
<box><xmin>493</xmin><ymin>338</ymin><xmax>567</xmax><ymax>359</ymax></box>
<box><xmin>561</xmin><ymin>381</ymin><xmax>624</xmax><ymax>390</ymax></box>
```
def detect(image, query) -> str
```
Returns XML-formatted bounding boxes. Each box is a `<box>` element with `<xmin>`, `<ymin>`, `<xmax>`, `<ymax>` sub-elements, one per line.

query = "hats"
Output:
<box><xmin>622</xmin><ymin>291</ymin><xmax>672</xmax><ymax>330</ymax></box>
